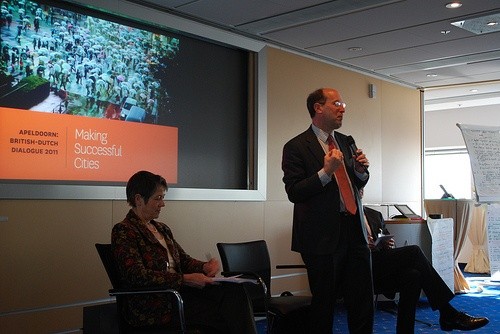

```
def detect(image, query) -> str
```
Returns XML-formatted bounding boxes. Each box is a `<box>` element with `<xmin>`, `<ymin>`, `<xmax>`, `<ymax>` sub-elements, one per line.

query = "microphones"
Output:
<box><xmin>347</xmin><ymin>135</ymin><xmax>370</xmax><ymax>175</ymax></box>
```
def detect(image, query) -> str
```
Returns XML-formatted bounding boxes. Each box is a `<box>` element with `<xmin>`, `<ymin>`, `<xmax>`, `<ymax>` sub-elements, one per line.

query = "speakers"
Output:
<box><xmin>370</xmin><ymin>84</ymin><xmax>377</xmax><ymax>98</ymax></box>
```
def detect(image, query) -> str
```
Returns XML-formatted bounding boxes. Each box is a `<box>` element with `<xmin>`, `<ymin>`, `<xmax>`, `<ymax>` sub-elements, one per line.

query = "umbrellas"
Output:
<box><xmin>1</xmin><ymin>1</ymin><xmax>155</xmax><ymax>104</ymax></box>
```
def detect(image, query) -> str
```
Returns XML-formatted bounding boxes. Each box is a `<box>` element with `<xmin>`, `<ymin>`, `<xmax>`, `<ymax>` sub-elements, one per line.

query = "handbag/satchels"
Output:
<box><xmin>264</xmin><ymin>290</ymin><xmax>314</xmax><ymax>334</ymax></box>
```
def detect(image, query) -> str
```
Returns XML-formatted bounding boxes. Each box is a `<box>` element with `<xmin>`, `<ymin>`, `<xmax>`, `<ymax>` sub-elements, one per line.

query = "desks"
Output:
<box><xmin>424</xmin><ymin>197</ymin><xmax>490</xmax><ymax>273</ymax></box>
<box><xmin>382</xmin><ymin>218</ymin><xmax>455</xmax><ymax>303</ymax></box>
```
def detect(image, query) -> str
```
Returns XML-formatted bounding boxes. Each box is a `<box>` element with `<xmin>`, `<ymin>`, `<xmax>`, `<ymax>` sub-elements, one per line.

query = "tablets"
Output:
<box><xmin>373</xmin><ymin>234</ymin><xmax>394</xmax><ymax>249</ymax></box>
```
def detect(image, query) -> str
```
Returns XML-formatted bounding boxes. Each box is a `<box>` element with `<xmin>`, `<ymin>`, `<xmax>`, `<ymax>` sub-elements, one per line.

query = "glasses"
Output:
<box><xmin>315</xmin><ymin>100</ymin><xmax>346</xmax><ymax>108</ymax></box>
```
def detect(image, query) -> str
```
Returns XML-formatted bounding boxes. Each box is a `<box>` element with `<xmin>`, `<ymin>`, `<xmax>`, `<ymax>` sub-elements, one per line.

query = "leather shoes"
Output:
<box><xmin>440</xmin><ymin>310</ymin><xmax>487</xmax><ymax>332</ymax></box>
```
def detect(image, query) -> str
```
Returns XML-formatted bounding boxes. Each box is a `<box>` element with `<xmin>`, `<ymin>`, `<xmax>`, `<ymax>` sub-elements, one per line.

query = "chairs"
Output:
<box><xmin>96</xmin><ymin>244</ymin><xmax>259</xmax><ymax>334</ymax></box>
<box><xmin>374</xmin><ymin>288</ymin><xmax>433</xmax><ymax>327</ymax></box>
<box><xmin>217</xmin><ymin>241</ymin><xmax>311</xmax><ymax>334</ymax></box>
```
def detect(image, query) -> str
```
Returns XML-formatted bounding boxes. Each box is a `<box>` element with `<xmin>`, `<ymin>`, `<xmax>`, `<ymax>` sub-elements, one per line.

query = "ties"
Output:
<box><xmin>364</xmin><ymin>211</ymin><xmax>375</xmax><ymax>249</ymax></box>
<box><xmin>327</xmin><ymin>133</ymin><xmax>359</xmax><ymax>214</ymax></box>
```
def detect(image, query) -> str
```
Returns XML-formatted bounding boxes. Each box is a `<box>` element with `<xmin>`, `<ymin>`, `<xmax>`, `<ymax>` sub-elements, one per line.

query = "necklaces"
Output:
<box><xmin>139</xmin><ymin>219</ymin><xmax>159</xmax><ymax>234</ymax></box>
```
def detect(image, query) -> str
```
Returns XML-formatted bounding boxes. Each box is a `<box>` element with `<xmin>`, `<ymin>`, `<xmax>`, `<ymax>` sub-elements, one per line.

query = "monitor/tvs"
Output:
<box><xmin>394</xmin><ymin>204</ymin><xmax>420</xmax><ymax>217</ymax></box>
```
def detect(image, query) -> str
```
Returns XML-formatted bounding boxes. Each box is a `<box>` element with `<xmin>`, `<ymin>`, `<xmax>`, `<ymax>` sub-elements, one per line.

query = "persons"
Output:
<box><xmin>111</xmin><ymin>170</ymin><xmax>220</xmax><ymax>334</ymax></box>
<box><xmin>282</xmin><ymin>88</ymin><xmax>375</xmax><ymax>334</ymax></box>
<box><xmin>0</xmin><ymin>3</ymin><xmax>180</xmax><ymax>110</ymax></box>
<box><xmin>358</xmin><ymin>183</ymin><xmax>490</xmax><ymax>334</ymax></box>
